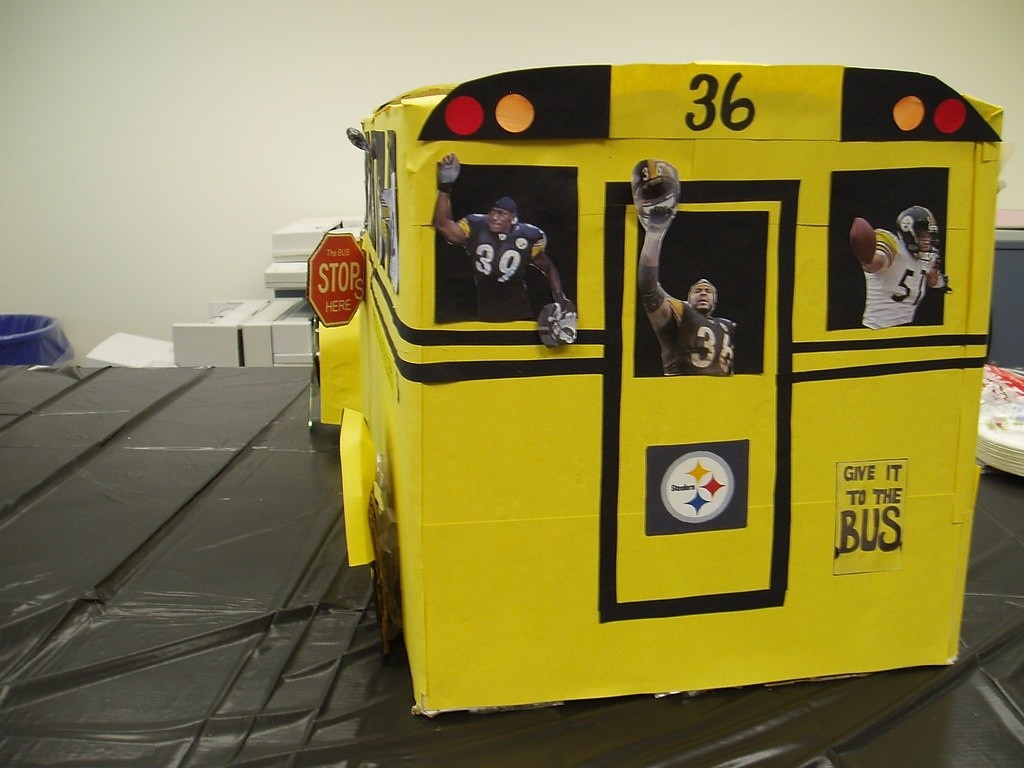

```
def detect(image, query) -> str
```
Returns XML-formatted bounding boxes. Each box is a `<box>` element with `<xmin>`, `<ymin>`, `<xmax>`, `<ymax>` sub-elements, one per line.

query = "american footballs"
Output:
<box><xmin>851</xmin><ymin>215</ymin><xmax>878</xmax><ymax>264</ymax></box>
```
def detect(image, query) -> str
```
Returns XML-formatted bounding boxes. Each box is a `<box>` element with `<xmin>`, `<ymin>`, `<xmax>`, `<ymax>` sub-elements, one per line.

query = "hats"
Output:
<box><xmin>493</xmin><ymin>196</ymin><xmax>518</xmax><ymax>217</ymax></box>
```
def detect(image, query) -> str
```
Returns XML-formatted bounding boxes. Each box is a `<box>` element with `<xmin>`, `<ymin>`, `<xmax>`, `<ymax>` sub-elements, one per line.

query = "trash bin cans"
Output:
<box><xmin>0</xmin><ymin>313</ymin><xmax>70</xmax><ymax>366</ymax></box>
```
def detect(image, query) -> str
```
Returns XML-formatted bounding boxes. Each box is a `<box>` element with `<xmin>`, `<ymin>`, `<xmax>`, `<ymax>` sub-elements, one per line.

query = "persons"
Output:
<box><xmin>632</xmin><ymin>159</ymin><xmax>737</xmax><ymax>377</ymax></box>
<box><xmin>431</xmin><ymin>153</ymin><xmax>577</xmax><ymax>321</ymax></box>
<box><xmin>850</xmin><ymin>205</ymin><xmax>951</xmax><ymax>331</ymax></box>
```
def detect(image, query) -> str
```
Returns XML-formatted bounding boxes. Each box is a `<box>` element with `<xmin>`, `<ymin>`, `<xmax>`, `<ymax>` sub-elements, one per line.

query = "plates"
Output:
<box><xmin>977</xmin><ymin>376</ymin><xmax>1024</xmax><ymax>477</ymax></box>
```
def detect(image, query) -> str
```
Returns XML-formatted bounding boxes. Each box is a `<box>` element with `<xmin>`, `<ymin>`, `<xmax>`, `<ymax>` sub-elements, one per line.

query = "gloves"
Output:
<box><xmin>646</xmin><ymin>211</ymin><xmax>678</xmax><ymax>230</ymax></box>
<box><xmin>436</xmin><ymin>154</ymin><xmax>461</xmax><ymax>193</ymax></box>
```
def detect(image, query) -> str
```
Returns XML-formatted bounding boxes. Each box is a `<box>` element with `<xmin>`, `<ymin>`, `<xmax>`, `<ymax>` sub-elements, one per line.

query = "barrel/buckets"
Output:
<box><xmin>0</xmin><ymin>313</ymin><xmax>59</xmax><ymax>365</ymax></box>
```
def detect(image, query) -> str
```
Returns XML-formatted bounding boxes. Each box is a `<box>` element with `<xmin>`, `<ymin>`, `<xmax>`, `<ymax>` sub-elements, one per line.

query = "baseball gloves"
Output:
<box><xmin>536</xmin><ymin>295</ymin><xmax>580</xmax><ymax>350</ymax></box>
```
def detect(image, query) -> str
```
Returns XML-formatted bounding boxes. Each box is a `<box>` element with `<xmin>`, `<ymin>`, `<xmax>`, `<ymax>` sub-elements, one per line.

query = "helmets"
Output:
<box><xmin>687</xmin><ymin>279</ymin><xmax>717</xmax><ymax>316</ymax></box>
<box><xmin>896</xmin><ymin>205</ymin><xmax>938</xmax><ymax>253</ymax></box>
<box><xmin>631</xmin><ymin>159</ymin><xmax>681</xmax><ymax>216</ymax></box>
